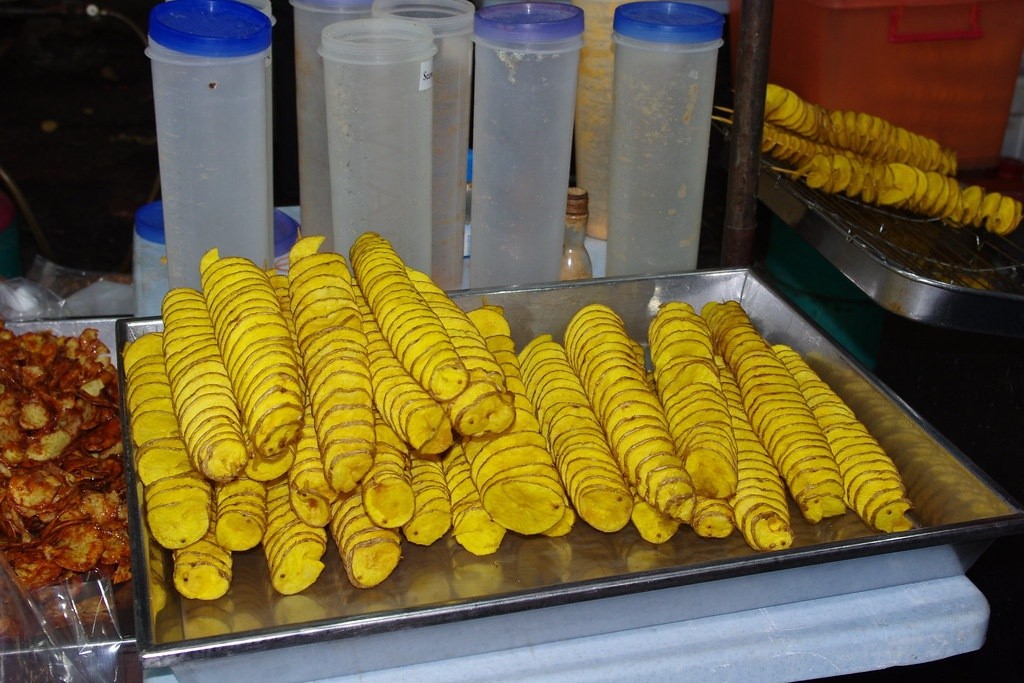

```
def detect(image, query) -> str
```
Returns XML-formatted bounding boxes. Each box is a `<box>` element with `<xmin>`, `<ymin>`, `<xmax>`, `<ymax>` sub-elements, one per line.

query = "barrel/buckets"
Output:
<box><xmin>133</xmin><ymin>203</ymin><xmax>301</xmax><ymax>317</ymax></box>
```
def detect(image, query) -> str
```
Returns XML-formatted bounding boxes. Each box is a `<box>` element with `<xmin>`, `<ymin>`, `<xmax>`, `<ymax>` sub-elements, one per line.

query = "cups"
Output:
<box><xmin>143</xmin><ymin>0</ymin><xmax>277</xmax><ymax>317</ymax></box>
<box><xmin>471</xmin><ymin>2</ymin><xmax>585</xmax><ymax>291</ymax></box>
<box><xmin>290</xmin><ymin>0</ymin><xmax>375</xmax><ymax>253</ymax></box>
<box><xmin>316</xmin><ymin>19</ymin><xmax>438</xmax><ymax>280</ymax></box>
<box><xmin>373</xmin><ymin>0</ymin><xmax>475</xmax><ymax>292</ymax></box>
<box><xmin>607</xmin><ymin>2</ymin><xmax>726</xmax><ymax>277</ymax></box>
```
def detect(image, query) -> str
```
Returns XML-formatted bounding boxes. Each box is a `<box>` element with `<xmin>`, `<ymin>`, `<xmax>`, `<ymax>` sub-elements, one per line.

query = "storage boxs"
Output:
<box><xmin>730</xmin><ymin>0</ymin><xmax>1024</xmax><ymax>173</ymax></box>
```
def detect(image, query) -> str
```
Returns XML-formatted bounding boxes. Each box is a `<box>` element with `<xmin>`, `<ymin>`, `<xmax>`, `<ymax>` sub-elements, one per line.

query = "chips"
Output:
<box><xmin>124</xmin><ymin>233</ymin><xmax>913</xmax><ymax>602</ymax></box>
<box><xmin>755</xmin><ymin>83</ymin><xmax>1023</xmax><ymax>236</ymax></box>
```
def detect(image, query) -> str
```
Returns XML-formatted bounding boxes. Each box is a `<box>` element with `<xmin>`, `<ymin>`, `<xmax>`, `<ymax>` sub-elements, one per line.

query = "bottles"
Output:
<box><xmin>561</xmin><ymin>188</ymin><xmax>592</xmax><ymax>281</ymax></box>
